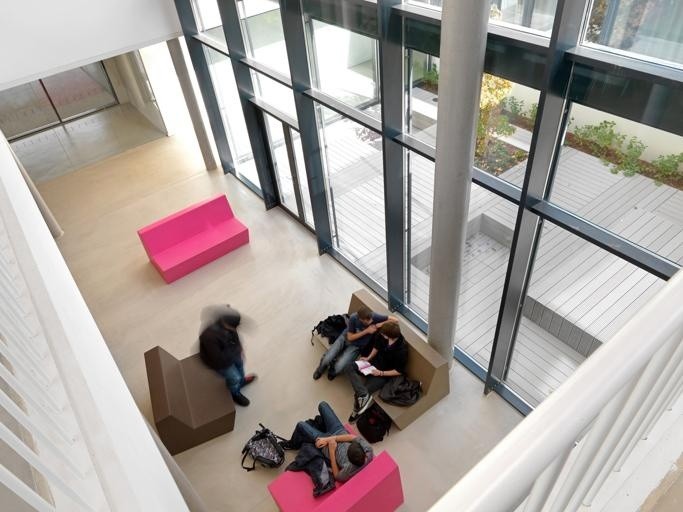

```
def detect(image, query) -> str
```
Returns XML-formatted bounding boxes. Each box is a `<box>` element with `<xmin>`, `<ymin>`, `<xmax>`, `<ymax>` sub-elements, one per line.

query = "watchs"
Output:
<box><xmin>381</xmin><ymin>371</ymin><xmax>384</xmax><ymax>376</ymax></box>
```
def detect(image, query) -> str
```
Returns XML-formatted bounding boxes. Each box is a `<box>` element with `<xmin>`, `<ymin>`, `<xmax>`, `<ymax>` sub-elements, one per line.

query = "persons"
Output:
<box><xmin>347</xmin><ymin>322</ymin><xmax>408</xmax><ymax>425</ymax></box>
<box><xmin>281</xmin><ymin>401</ymin><xmax>373</xmax><ymax>483</ymax></box>
<box><xmin>199</xmin><ymin>305</ymin><xmax>257</xmax><ymax>407</ymax></box>
<box><xmin>313</xmin><ymin>306</ymin><xmax>399</xmax><ymax>381</ymax></box>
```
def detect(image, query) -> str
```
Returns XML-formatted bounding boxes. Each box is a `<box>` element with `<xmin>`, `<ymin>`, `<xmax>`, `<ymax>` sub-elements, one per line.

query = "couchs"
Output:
<box><xmin>144</xmin><ymin>345</ymin><xmax>236</xmax><ymax>457</ymax></box>
<box><xmin>266</xmin><ymin>423</ymin><xmax>404</xmax><ymax>512</ymax></box>
<box><xmin>137</xmin><ymin>192</ymin><xmax>250</xmax><ymax>284</ymax></box>
<box><xmin>312</xmin><ymin>287</ymin><xmax>450</xmax><ymax>431</ymax></box>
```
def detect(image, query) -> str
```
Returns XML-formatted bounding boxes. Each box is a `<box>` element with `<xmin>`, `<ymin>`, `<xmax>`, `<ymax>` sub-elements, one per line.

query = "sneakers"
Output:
<box><xmin>313</xmin><ymin>367</ymin><xmax>323</xmax><ymax>380</ymax></box>
<box><xmin>357</xmin><ymin>392</ymin><xmax>373</xmax><ymax>416</ymax></box>
<box><xmin>232</xmin><ymin>392</ymin><xmax>250</xmax><ymax>406</ymax></box>
<box><xmin>347</xmin><ymin>409</ymin><xmax>363</xmax><ymax>425</ymax></box>
<box><xmin>327</xmin><ymin>366</ymin><xmax>335</xmax><ymax>380</ymax></box>
<box><xmin>277</xmin><ymin>439</ymin><xmax>301</xmax><ymax>454</ymax></box>
<box><xmin>239</xmin><ymin>373</ymin><xmax>256</xmax><ymax>388</ymax></box>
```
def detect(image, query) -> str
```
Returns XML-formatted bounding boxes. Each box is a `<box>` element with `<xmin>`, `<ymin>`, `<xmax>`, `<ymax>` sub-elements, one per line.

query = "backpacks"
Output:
<box><xmin>241</xmin><ymin>423</ymin><xmax>289</xmax><ymax>473</ymax></box>
<box><xmin>357</xmin><ymin>403</ymin><xmax>393</xmax><ymax>444</ymax></box>
<box><xmin>311</xmin><ymin>315</ymin><xmax>347</xmax><ymax>345</ymax></box>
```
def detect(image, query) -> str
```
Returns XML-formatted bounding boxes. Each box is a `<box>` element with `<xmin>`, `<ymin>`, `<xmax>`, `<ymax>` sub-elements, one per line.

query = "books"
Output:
<box><xmin>355</xmin><ymin>360</ymin><xmax>377</xmax><ymax>376</ymax></box>
